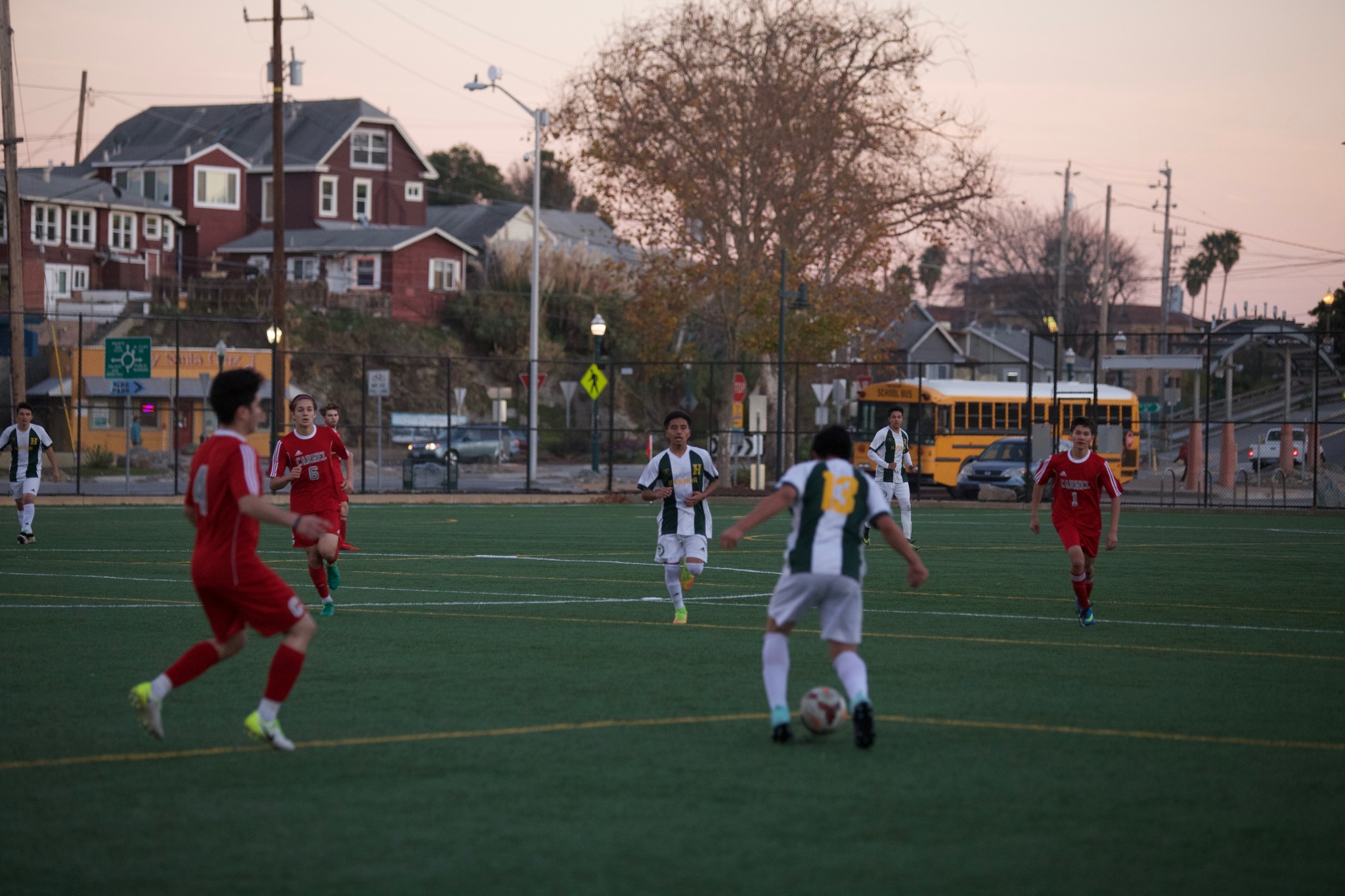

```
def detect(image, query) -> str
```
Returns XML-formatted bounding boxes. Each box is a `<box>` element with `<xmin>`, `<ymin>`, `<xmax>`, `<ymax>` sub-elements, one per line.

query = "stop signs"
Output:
<box><xmin>1126</xmin><ymin>432</ymin><xmax>1135</xmax><ymax>451</ymax></box>
<box><xmin>732</xmin><ymin>372</ymin><xmax>748</xmax><ymax>403</ymax></box>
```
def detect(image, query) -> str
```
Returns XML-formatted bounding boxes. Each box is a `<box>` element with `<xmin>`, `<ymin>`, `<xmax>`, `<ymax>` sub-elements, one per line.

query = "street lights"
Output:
<box><xmin>266</xmin><ymin>321</ymin><xmax>283</xmax><ymax>457</ymax></box>
<box><xmin>590</xmin><ymin>312</ymin><xmax>608</xmax><ymax>473</ymax></box>
<box><xmin>215</xmin><ymin>338</ymin><xmax>226</xmax><ymax>375</ymax></box>
<box><xmin>462</xmin><ymin>63</ymin><xmax>550</xmax><ymax>491</ymax></box>
<box><xmin>775</xmin><ymin>248</ymin><xmax>812</xmax><ymax>479</ymax></box>
<box><xmin>1113</xmin><ymin>330</ymin><xmax>1128</xmax><ymax>388</ymax></box>
<box><xmin>1064</xmin><ymin>347</ymin><xmax>1076</xmax><ymax>382</ymax></box>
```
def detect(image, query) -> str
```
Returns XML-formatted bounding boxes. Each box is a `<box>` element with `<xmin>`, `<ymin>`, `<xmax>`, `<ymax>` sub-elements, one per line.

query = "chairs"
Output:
<box><xmin>1008</xmin><ymin>447</ymin><xmax>1022</xmax><ymax>462</ymax></box>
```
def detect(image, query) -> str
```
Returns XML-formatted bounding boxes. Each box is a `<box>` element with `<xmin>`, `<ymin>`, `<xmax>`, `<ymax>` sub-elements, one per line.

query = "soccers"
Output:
<box><xmin>799</xmin><ymin>686</ymin><xmax>846</xmax><ymax>731</ymax></box>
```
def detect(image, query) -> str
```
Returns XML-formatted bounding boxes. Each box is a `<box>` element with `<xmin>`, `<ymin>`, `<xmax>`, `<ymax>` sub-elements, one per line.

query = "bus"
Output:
<box><xmin>839</xmin><ymin>377</ymin><xmax>1141</xmax><ymax>498</ymax></box>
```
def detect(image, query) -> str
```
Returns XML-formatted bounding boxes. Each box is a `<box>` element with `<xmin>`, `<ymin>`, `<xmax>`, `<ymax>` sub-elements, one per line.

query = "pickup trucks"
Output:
<box><xmin>1248</xmin><ymin>427</ymin><xmax>1326</xmax><ymax>473</ymax></box>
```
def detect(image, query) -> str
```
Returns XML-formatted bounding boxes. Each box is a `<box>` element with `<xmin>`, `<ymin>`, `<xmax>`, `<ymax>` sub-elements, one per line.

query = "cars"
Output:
<box><xmin>956</xmin><ymin>437</ymin><xmax>1075</xmax><ymax>501</ymax></box>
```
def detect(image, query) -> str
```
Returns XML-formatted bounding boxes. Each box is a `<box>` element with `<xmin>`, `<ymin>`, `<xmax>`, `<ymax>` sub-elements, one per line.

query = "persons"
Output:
<box><xmin>0</xmin><ymin>402</ymin><xmax>60</xmax><ymax>547</ymax></box>
<box><xmin>127</xmin><ymin>367</ymin><xmax>316</xmax><ymax>750</ymax></box>
<box><xmin>719</xmin><ymin>427</ymin><xmax>928</xmax><ymax>749</ymax></box>
<box><xmin>268</xmin><ymin>394</ymin><xmax>360</xmax><ymax>617</ymax></box>
<box><xmin>636</xmin><ymin>412</ymin><xmax>721</xmax><ymax>626</ymax></box>
<box><xmin>1177</xmin><ymin>437</ymin><xmax>1189</xmax><ymax>486</ymax></box>
<box><xmin>862</xmin><ymin>406</ymin><xmax>918</xmax><ymax>549</ymax></box>
<box><xmin>131</xmin><ymin>416</ymin><xmax>143</xmax><ymax>446</ymax></box>
<box><xmin>1031</xmin><ymin>416</ymin><xmax>1121</xmax><ymax>628</ymax></box>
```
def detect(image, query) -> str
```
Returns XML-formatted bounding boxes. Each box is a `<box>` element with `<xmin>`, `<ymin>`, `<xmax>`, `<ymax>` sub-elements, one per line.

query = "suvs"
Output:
<box><xmin>404</xmin><ymin>424</ymin><xmax>519</xmax><ymax>466</ymax></box>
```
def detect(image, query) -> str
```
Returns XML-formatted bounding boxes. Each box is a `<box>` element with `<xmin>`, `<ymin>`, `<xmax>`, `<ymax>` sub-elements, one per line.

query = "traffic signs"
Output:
<box><xmin>108</xmin><ymin>379</ymin><xmax>145</xmax><ymax>397</ymax></box>
<box><xmin>102</xmin><ymin>335</ymin><xmax>152</xmax><ymax>381</ymax></box>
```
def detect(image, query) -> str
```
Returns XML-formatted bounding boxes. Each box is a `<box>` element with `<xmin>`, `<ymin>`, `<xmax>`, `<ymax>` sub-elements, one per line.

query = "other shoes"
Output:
<box><xmin>850</xmin><ymin>701</ymin><xmax>876</xmax><ymax>748</ymax></box>
<box><xmin>18</xmin><ymin>536</ymin><xmax>35</xmax><ymax>544</ymax></box>
<box><xmin>772</xmin><ymin>724</ymin><xmax>792</xmax><ymax>742</ymax></box>
<box><xmin>17</xmin><ymin>535</ymin><xmax>26</xmax><ymax>544</ymax></box>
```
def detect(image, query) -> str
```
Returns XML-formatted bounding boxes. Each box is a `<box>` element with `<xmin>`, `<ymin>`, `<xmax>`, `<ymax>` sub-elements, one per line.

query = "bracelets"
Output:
<box><xmin>291</xmin><ymin>514</ymin><xmax>304</xmax><ymax>531</ymax></box>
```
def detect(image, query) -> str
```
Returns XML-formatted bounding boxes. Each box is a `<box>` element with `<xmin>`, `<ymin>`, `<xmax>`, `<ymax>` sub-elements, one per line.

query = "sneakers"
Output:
<box><xmin>908</xmin><ymin>540</ymin><xmax>920</xmax><ymax>551</ymax></box>
<box><xmin>673</xmin><ymin>609</ymin><xmax>688</xmax><ymax>625</ymax></box>
<box><xmin>862</xmin><ymin>534</ymin><xmax>870</xmax><ymax>545</ymax></box>
<box><xmin>679</xmin><ymin>558</ymin><xmax>694</xmax><ymax>590</ymax></box>
<box><xmin>319</xmin><ymin>603</ymin><xmax>335</xmax><ymax>617</ymax></box>
<box><xmin>246</xmin><ymin>712</ymin><xmax>295</xmax><ymax>750</ymax></box>
<box><xmin>128</xmin><ymin>682</ymin><xmax>163</xmax><ymax>738</ymax></box>
<box><xmin>326</xmin><ymin>563</ymin><xmax>340</xmax><ymax>591</ymax></box>
<box><xmin>1075</xmin><ymin>598</ymin><xmax>1094</xmax><ymax>627</ymax></box>
<box><xmin>339</xmin><ymin>543</ymin><xmax>359</xmax><ymax>551</ymax></box>
